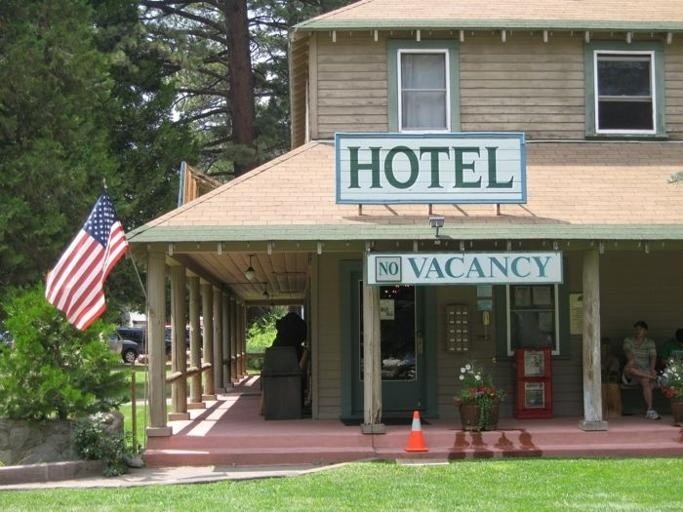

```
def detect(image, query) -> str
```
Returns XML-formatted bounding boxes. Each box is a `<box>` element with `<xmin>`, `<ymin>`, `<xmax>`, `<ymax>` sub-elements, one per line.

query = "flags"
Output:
<box><xmin>45</xmin><ymin>190</ymin><xmax>130</xmax><ymax>333</ymax></box>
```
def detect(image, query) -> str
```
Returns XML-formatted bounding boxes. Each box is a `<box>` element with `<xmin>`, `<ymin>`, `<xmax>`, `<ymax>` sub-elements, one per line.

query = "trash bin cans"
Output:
<box><xmin>261</xmin><ymin>346</ymin><xmax>304</xmax><ymax>420</ymax></box>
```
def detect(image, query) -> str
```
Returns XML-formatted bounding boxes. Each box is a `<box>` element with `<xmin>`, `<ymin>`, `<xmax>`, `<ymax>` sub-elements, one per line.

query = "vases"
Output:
<box><xmin>670</xmin><ymin>401</ymin><xmax>682</xmax><ymax>422</ymax></box>
<box><xmin>459</xmin><ymin>402</ymin><xmax>500</xmax><ymax>431</ymax></box>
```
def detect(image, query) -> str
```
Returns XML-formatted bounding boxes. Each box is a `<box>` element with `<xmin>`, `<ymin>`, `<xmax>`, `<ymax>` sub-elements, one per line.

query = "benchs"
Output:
<box><xmin>604</xmin><ymin>380</ymin><xmax>667</xmax><ymax>417</ymax></box>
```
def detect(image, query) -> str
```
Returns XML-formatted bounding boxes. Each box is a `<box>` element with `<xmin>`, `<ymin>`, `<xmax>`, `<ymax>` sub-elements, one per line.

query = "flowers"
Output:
<box><xmin>658</xmin><ymin>359</ymin><xmax>683</xmax><ymax>400</ymax></box>
<box><xmin>453</xmin><ymin>357</ymin><xmax>507</xmax><ymax>409</ymax></box>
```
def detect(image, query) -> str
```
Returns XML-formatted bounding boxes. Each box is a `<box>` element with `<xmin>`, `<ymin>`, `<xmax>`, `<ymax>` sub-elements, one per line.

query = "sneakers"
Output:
<box><xmin>646</xmin><ymin>409</ymin><xmax>659</xmax><ymax>420</ymax></box>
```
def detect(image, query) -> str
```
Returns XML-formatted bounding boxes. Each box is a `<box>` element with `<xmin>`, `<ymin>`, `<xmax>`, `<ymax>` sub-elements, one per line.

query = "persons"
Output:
<box><xmin>602</xmin><ymin>320</ymin><xmax>683</xmax><ymax>421</ymax></box>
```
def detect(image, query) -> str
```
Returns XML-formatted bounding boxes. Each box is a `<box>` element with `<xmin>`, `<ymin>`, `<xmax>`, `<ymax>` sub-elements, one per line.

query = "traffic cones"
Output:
<box><xmin>404</xmin><ymin>410</ymin><xmax>429</xmax><ymax>452</ymax></box>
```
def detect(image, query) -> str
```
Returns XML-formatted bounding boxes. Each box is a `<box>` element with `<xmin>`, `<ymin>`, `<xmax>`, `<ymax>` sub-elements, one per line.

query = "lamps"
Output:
<box><xmin>261</xmin><ymin>281</ymin><xmax>275</xmax><ymax>310</ymax></box>
<box><xmin>244</xmin><ymin>253</ymin><xmax>256</xmax><ymax>281</ymax></box>
<box><xmin>427</xmin><ymin>215</ymin><xmax>446</xmax><ymax>237</ymax></box>
